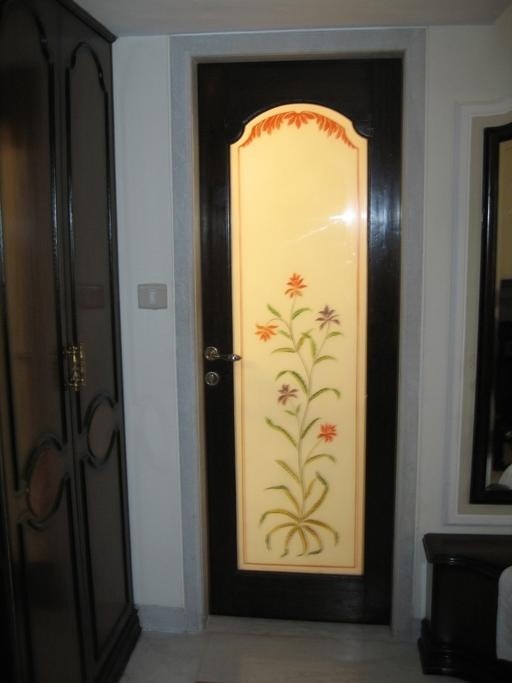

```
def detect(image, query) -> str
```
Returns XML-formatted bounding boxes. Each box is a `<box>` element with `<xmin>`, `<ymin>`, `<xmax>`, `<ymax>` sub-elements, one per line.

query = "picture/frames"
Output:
<box><xmin>443</xmin><ymin>98</ymin><xmax>512</xmax><ymax>526</ymax></box>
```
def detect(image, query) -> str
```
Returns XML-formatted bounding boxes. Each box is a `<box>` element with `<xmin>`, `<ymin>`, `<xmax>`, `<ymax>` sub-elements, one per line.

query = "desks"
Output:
<box><xmin>418</xmin><ymin>532</ymin><xmax>511</xmax><ymax>682</ymax></box>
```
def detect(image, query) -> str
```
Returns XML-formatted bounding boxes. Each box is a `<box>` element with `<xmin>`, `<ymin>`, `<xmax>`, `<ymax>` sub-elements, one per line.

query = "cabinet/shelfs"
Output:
<box><xmin>0</xmin><ymin>1</ymin><xmax>141</xmax><ymax>683</ymax></box>
